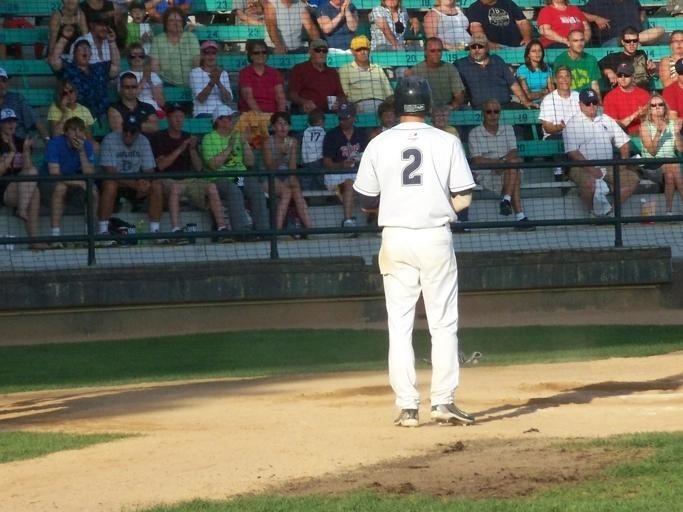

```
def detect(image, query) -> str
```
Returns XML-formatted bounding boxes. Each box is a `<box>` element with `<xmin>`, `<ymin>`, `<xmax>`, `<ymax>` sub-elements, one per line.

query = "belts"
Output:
<box><xmin>354</xmin><ymin>98</ymin><xmax>387</xmax><ymax>104</ymax></box>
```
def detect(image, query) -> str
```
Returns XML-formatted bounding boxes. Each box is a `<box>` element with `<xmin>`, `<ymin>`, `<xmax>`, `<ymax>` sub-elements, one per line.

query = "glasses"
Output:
<box><xmin>483</xmin><ymin>109</ymin><xmax>501</xmax><ymax>114</ymax></box>
<box><xmin>428</xmin><ymin>48</ymin><xmax>443</xmax><ymax>53</ymax></box>
<box><xmin>310</xmin><ymin>48</ymin><xmax>328</xmax><ymax>53</ymax></box>
<box><xmin>469</xmin><ymin>44</ymin><xmax>486</xmax><ymax>49</ymax></box>
<box><xmin>582</xmin><ymin>100</ymin><xmax>599</xmax><ymax>107</ymax></box>
<box><xmin>650</xmin><ymin>103</ymin><xmax>664</xmax><ymax>108</ymax></box>
<box><xmin>250</xmin><ymin>48</ymin><xmax>269</xmax><ymax>55</ymax></box>
<box><xmin>669</xmin><ymin>39</ymin><xmax>683</xmax><ymax>43</ymax></box>
<box><xmin>622</xmin><ymin>38</ymin><xmax>638</xmax><ymax>43</ymax></box>
<box><xmin>615</xmin><ymin>73</ymin><xmax>632</xmax><ymax>78</ymax></box>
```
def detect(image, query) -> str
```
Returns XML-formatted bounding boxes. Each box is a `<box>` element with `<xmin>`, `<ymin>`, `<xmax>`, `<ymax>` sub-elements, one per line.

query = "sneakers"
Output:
<box><xmin>393</xmin><ymin>409</ymin><xmax>419</xmax><ymax>427</ymax></box>
<box><xmin>51</xmin><ymin>242</ymin><xmax>64</xmax><ymax>249</ymax></box>
<box><xmin>157</xmin><ymin>239</ymin><xmax>170</xmax><ymax>244</ymax></box>
<box><xmin>174</xmin><ymin>230</ymin><xmax>188</xmax><ymax>245</ymax></box>
<box><xmin>95</xmin><ymin>232</ymin><xmax>117</xmax><ymax>248</ymax></box>
<box><xmin>500</xmin><ymin>199</ymin><xmax>512</xmax><ymax>216</ymax></box>
<box><xmin>430</xmin><ymin>403</ymin><xmax>474</xmax><ymax>425</ymax></box>
<box><xmin>217</xmin><ymin>229</ymin><xmax>233</xmax><ymax>243</ymax></box>
<box><xmin>342</xmin><ymin>218</ymin><xmax>358</xmax><ymax>238</ymax></box>
<box><xmin>514</xmin><ymin>216</ymin><xmax>536</xmax><ymax>231</ymax></box>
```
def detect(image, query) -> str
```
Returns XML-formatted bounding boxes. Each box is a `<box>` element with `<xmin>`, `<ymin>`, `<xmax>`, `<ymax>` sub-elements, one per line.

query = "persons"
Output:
<box><xmin>0</xmin><ymin>0</ymin><xmax>683</xmax><ymax>250</ymax></box>
<box><xmin>351</xmin><ymin>75</ymin><xmax>477</xmax><ymax>428</ymax></box>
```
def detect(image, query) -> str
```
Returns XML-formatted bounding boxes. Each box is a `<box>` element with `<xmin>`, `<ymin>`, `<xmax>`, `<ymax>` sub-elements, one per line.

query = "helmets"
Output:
<box><xmin>394</xmin><ymin>76</ymin><xmax>433</xmax><ymax>116</ymax></box>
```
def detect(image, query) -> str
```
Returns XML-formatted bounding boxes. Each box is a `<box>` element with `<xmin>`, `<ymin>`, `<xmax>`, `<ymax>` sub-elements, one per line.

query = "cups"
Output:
<box><xmin>5</xmin><ymin>235</ymin><xmax>16</xmax><ymax>250</ymax></box>
<box><xmin>327</xmin><ymin>96</ymin><xmax>337</xmax><ymax>110</ymax></box>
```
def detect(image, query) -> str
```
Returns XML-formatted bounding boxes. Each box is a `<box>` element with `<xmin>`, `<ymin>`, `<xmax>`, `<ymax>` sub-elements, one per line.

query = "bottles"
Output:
<box><xmin>136</xmin><ymin>218</ymin><xmax>144</xmax><ymax>247</ymax></box>
<box><xmin>293</xmin><ymin>219</ymin><xmax>301</xmax><ymax>239</ymax></box>
<box><xmin>555</xmin><ymin>167</ymin><xmax>563</xmax><ymax>182</ymax></box>
<box><xmin>187</xmin><ymin>223</ymin><xmax>197</xmax><ymax>243</ymax></box>
<box><xmin>464</xmin><ymin>43</ymin><xmax>470</xmax><ymax>50</ymax></box>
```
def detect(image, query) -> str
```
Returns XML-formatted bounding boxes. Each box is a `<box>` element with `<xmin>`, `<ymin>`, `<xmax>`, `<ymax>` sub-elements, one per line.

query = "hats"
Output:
<box><xmin>338</xmin><ymin>101</ymin><xmax>357</xmax><ymax>119</ymax></box>
<box><xmin>1</xmin><ymin>1</ymin><xmax>237</xmax><ymax>134</ymax></box>
<box><xmin>310</xmin><ymin>39</ymin><xmax>328</xmax><ymax>49</ymax></box>
<box><xmin>675</xmin><ymin>58</ymin><xmax>683</xmax><ymax>74</ymax></box>
<box><xmin>351</xmin><ymin>34</ymin><xmax>370</xmax><ymax>50</ymax></box>
